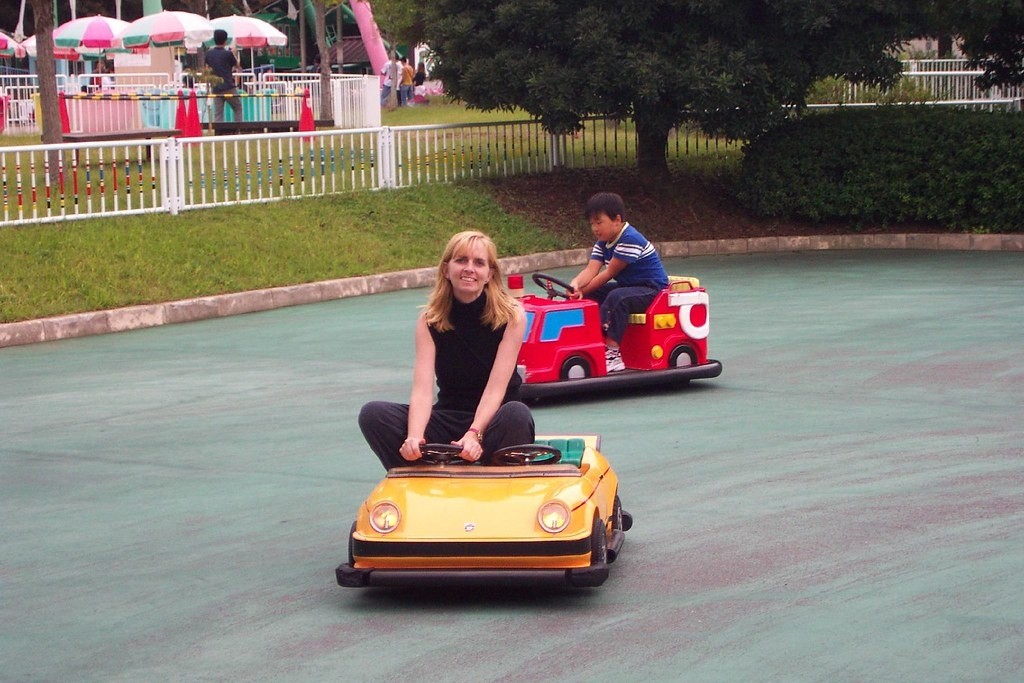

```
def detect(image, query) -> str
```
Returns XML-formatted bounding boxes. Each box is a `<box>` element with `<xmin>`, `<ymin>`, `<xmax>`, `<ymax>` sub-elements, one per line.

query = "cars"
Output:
<box><xmin>508</xmin><ymin>272</ymin><xmax>723</xmax><ymax>399</ymax></box>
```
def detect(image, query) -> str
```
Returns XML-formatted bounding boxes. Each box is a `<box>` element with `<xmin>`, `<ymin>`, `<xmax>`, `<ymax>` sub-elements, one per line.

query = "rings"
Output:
<box><xmin>403</xmin><ymin>456</ymin><xmax>406</xmax><ymax>459</ymax></box>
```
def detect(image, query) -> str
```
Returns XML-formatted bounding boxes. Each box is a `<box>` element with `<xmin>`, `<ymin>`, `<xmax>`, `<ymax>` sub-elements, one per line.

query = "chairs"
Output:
<box><xmin>534</xmin><ymin>439</ymin><xmax>585</xmax><ymax>467</ymax></box>
<box><xmin>629</xmin><ymin>275</ymin><xmax>699</xmax><ymax>326</ymax></box>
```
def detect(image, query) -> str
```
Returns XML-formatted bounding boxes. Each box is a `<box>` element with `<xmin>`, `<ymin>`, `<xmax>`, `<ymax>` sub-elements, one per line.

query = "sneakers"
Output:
<box><xmin>604</xmin><ymin>347</ymin><xmax>625</xmax><ymax>373</ymax></box>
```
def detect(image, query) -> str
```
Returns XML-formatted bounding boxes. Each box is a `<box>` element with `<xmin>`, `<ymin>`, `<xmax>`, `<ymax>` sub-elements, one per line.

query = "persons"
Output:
<box><xmin>562</xmin><ymin>192</ymin><xmax>669</xmax><ymax>373</ymax></box>
<box><xmin>357</xmin><ymin>231</ymin><xmax>535</xmax><ymax>480</ymax></box>
<box><xmin>204</xmin><ymin>29</ymin><xmax>241</xmax><ymax>124</ymax></box>
<box><xmin>381</xmin><ymin>51</ymin><xmax>426</xmax><ymax>115</ymax></box>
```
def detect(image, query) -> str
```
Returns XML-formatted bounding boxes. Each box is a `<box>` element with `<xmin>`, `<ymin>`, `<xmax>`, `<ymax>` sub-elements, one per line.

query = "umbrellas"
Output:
<box><xmin>0</xmin><ymin>10</ymin><xmax>287</xmax><ymax>138</ymax></box>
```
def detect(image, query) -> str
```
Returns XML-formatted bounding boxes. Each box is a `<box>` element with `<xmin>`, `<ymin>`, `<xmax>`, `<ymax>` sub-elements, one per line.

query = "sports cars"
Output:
<box><xmin>334</xmin><ymin>429</ymin><xmax>632</xmax><ymax>587</ymax></box>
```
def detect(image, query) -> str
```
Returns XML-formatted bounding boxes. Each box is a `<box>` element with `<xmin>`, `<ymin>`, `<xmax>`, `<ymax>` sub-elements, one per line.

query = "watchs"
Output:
<box><xmin>467</xmin><ymin>428</ymin><xmax>483</xmax><ymax>444</ymax></box>
